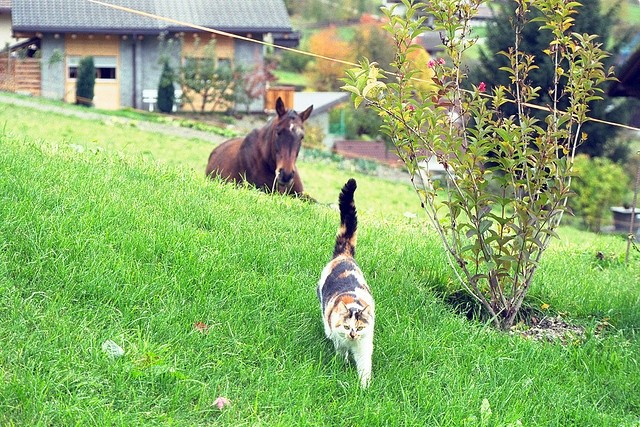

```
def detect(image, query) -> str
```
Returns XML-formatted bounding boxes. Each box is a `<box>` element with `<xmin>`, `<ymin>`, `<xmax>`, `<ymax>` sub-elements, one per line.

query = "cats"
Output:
<box><xmin>317</xmin><ymin>179</ymin><xmax>376</xmax><ymax>390</ymax></box>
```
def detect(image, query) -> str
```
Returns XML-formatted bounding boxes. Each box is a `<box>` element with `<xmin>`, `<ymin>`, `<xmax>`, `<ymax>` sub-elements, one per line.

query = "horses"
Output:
<box><xmin>206</xmin><ymin>97</ymin><xmax>316</xmax><ymax>205</ymax></box>
